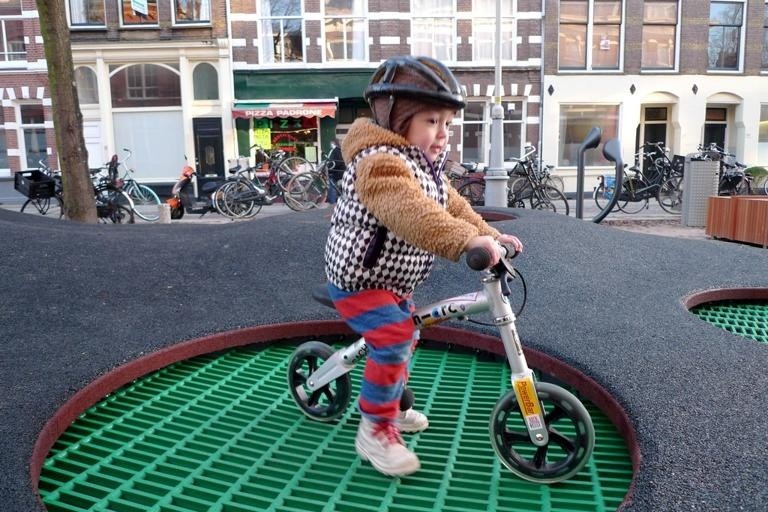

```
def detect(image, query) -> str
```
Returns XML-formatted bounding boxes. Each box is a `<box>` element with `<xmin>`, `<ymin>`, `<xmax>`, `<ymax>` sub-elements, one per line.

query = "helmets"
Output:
<box><xmin>364</xmin><ymin>54</ymin><xmax>465</xmax><ymax>134</ymax></box>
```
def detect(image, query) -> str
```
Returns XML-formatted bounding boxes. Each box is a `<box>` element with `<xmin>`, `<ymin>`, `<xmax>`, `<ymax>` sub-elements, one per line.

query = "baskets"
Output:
<box><xmin>15</xmin><ymin>169</ymin><xmax>55</xmax><ymax>199</ymax></box>
<box><xmin>670</xmin><ymin>155</ymin><xmax>684</xmax><ymax>172</ymax></box>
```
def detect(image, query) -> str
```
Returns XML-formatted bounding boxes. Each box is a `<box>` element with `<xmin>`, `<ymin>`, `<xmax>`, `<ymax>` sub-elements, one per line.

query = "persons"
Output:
<box><xmin>324</xmin><ymin>55</ymin><xmax>522</xmax><ymax>477</ymax></box>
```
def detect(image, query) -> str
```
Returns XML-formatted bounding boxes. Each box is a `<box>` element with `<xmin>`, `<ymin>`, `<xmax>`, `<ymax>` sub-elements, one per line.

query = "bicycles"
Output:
<box><xmin>288</xmin><ymin>242</ymin><xmax>595</xmax><ymax>485</ymax></box>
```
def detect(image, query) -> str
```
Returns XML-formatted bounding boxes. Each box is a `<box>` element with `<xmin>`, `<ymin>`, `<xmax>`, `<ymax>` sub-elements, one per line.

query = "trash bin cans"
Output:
<box><xmin>681</xmin><ymin>151</ymin><xmax>720</xmax><ymax>228</ymax></box>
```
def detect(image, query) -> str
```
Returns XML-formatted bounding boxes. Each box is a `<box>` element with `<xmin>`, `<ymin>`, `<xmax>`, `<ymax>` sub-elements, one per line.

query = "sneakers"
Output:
<box><xmin>355</xmin><ymin>406</ymin><xmax>428</xmax><ymax>477</ymax></box>
